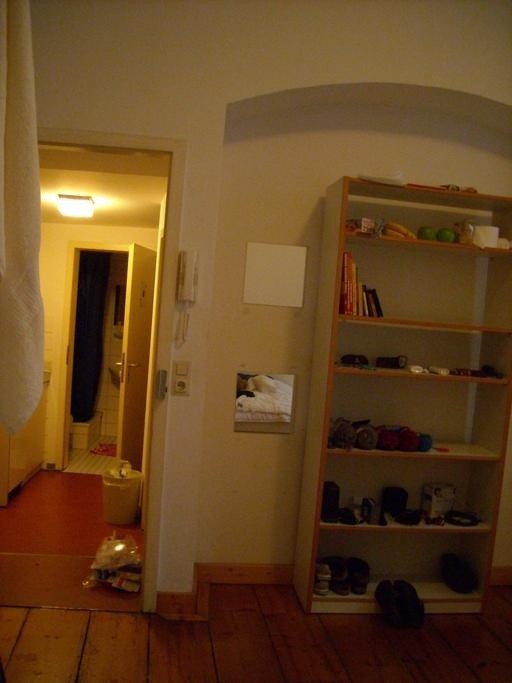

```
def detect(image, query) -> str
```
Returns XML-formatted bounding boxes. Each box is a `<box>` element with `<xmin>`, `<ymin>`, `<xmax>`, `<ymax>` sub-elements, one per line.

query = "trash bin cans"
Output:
<box><xmin>102</xmin><ymin>467</ymin><xmax>142</xmax><ymax>525</ymax></box>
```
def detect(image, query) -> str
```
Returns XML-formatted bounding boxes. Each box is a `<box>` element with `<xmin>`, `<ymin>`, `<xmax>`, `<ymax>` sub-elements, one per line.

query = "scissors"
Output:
<box><xmin>432</xmin><ymin>446</ymin><xmax>449</xmax><ymax>452</ymax></box>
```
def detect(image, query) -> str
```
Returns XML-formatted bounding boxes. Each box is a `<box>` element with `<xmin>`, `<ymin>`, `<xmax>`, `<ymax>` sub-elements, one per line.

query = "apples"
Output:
<box><xmin>438</xmin><ymin>228</ymin><xmax>456</xmax><ymax>243</ymax></box>
<box><xmin>418</xmin><ymin>227</ymin><xmax>439</xmax><ymax>240</ymax></box>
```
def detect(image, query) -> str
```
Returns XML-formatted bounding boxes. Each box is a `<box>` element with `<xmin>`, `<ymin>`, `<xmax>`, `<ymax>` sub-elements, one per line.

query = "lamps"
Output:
<box><xmin>57</xmin><ymin>193</ymin><xmax>95</xmax><ymax>219</ymax></box>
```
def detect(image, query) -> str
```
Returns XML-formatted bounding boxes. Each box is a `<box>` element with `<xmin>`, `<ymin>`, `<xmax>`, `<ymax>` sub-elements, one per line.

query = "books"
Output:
<box><xmin>341</xmin><ymin>251</ymin><xmax>382</xmax><ymax>319</ymax></box>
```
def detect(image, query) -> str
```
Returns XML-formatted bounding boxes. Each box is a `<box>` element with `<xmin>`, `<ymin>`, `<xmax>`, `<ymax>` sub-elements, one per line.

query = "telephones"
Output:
<box><xmin>177</xmin><ymin>250</ymin><xmax>198</xmax><ymax>303</ymax></box>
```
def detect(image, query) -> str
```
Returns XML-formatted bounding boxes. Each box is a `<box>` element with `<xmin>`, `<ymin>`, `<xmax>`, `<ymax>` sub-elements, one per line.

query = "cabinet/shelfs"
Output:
<box><xmin>292</xmin><ymin>176</ymin><xmax>512</xmax><ymax>615</ymax></box>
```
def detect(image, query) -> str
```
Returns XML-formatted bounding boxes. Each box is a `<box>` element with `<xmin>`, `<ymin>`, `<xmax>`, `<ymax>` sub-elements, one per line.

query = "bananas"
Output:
<box><xmin>382</xmin><ymin>223</ymin><xmax>416</xmax><ymax>239</ymax></box>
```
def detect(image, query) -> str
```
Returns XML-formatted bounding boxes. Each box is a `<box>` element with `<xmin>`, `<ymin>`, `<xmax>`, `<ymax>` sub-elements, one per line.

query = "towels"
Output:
<box><xmin>0</xmin><ymin>0</ymin><xmax>46</xmax><ymax>435</ymax></box>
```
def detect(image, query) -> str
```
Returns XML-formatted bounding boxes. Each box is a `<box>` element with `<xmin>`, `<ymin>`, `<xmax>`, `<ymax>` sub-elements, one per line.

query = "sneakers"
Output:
<box><xmin>313</xmin><ymin>557</ymin><xmax>424</xmax><ymax>629</ymax></box>
